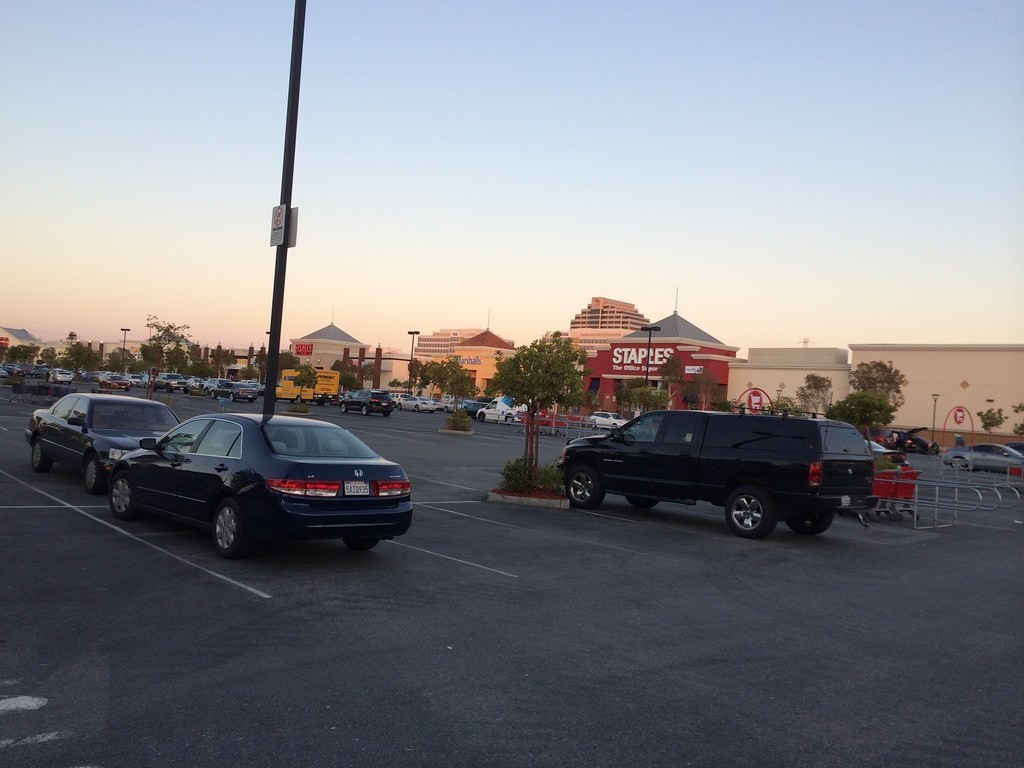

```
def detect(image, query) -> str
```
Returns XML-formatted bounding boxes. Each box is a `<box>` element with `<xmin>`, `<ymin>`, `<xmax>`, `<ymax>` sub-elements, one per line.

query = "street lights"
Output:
<box><xmin>640</xmin><ymin>326</ymin><xmax>661</xmax><ymax>388</ymax></box>
<box><xmin>931</xmin><ymin>393</ymin><xmax>939</xmax><ymax>443</ymax></box>
<box><xmin>408</xmin><ymin>331</ymin><xmax>421</xmax><ymax>392</ymax></box>
<box><xmin>121</xmin><ymin>328</ymin><xmax>130</xmax><ymax>357</ymax></box>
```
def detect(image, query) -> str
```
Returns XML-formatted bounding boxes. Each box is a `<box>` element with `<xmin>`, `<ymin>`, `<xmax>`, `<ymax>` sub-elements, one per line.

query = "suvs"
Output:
<box><xmin>339</xmin><ymin>390</ymin><xmax>397</xmax><ymax>417</ymax></box>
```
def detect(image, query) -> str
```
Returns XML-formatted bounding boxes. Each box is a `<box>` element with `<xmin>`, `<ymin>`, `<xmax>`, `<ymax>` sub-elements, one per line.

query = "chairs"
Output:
<box><xmin>272</xmin><ymin>441</ymin><xmax>289</xmax><ymax>455</ymax></box>
<box><xmin>93</xmin><ymin>411</ymin><xmax>108</xmax><ymax>428</ymax></box>
<box><xmin>219</xmin><ymin>433</ymin><xmax>240</xmax><ymax>456</ymax></box>
<box><xmin>322</xmin><ymin>440</ymin><xmax>346</xmax><ymax>457</ymax></box>
<box><xmin>112</xmin><ymin>411</ymin><xmax>134</xmax><ymax>429</ymax></box>
<box><xmin>274</xmin><ymin>431</ymin><xmax>299</xmax><ymax>448</ymax></box>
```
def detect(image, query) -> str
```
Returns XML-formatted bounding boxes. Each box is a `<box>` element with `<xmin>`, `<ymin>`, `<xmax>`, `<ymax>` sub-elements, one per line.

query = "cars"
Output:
<box><xmin>589</xmin><ymin>412</ymin><xmax>629</xmax><ymax>430</ymax></box>
<box><xmin>51</xmin><ymin>369</ymin><xmax>72</xmax><ymax>385</ymax></box>
<box><xmin>942</xmin><ymin>444</ymin><xmax>1024</xmax><ymax>473</ymax></box>
<box><xmin>208</xmin><ymin>382</ymin><xmax>258</xmax><ymax>402</ymax></box>
<box><xmin>397</xmin><ymin>396</ymin><xmax>436</xmax><ymax>413</ymax></box>
<box><xmin>430</xmin><ymin>393</ymin><xmax>497</xmax><ymax>413</ymax></box>
<box><xmin>462</xmin><ymin>401</ymin><xmax>489</xmax><ymax>419</ymax></box>
<box><xmin>98</xmin><ymin>373</ymin><xmax>131</xmax><ymax>392</ymax></box>
<box><xmin>106</xmin><ymin>412</ymin><xmax>414</xmax><ymax>561</ymax></box>
<box><xmin>0</xmin><ymin>362</ymin><xmax>266</xmax><ymax>397</ymax></box>
<box><xmin>863</xmin><ymin>439</ymin><xmax>911</xmax><ymax>469</ymax></box>
<box><xmin>24</xmin><ymin>392</ymin><xmax>182</xmax><ymax>497</ymax></box>
<box><xmin>1005</xmin><ymin>443</ymin><xmax>1024</xmax><ymax>454</ymax></box>
<box><xmin>390</xmin><ymin>393</ymin><xmax>411</xmax><ymax>407</ymax></box>
<box><xmin>878</xmin><ymin>426</ymin><xmax>929</xmax><ymax>453</ymax></box>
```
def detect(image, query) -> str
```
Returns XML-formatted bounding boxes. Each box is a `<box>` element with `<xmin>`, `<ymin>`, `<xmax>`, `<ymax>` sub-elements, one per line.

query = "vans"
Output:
<box><xmin>477</xmin><ymin>397</ymin><xmax>528</xmax><ymax>425</ymax></box>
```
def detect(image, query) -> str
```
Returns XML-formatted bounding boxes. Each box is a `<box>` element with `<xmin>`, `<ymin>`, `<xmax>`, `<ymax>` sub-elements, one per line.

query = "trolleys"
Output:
<box><xmin>856</xmin><ymin>469</ymin><xmax>923</xmax><ymax>528</ymax></box>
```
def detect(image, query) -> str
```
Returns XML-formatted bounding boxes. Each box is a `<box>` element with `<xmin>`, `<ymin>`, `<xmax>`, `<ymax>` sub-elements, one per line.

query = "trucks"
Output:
<box><xmin>276</xmin><ymin>368</ymin><xmax>340</xmax><ymax>407</ymax></box>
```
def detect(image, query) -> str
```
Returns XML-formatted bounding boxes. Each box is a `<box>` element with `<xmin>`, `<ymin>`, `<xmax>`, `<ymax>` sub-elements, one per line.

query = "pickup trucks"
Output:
<box><xmin>149</xmin><ymin>373</ymin><xmax>189</xmax><ymax>394</ymax></box>
<box><xmin>555</xmin><ymin>408</ymin><xmax>880</xmax><ymax>539</ymax></box>
